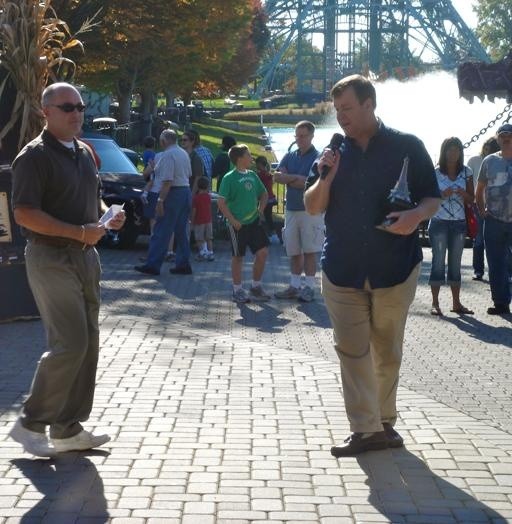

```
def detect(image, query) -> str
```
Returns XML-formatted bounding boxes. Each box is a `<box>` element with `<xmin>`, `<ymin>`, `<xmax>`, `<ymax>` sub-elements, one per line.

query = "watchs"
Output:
<box><xmin>157</xmin><ymin>198</ymin><xmax>164</xmax><ymax>202</ymax></box>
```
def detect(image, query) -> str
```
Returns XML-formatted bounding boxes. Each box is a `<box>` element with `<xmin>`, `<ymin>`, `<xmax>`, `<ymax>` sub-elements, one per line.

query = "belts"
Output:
<box><xmin>26</xmin><ymin>239</ymin><xmax>93</xmax><ymax>252</ymax></box>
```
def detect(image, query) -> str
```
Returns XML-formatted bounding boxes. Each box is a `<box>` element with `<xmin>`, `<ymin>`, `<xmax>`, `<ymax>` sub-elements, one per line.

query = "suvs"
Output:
<box><xmin>83</xmin><ymin>137</ymin><xmax>152</xmax><ymax>251</ymax></box>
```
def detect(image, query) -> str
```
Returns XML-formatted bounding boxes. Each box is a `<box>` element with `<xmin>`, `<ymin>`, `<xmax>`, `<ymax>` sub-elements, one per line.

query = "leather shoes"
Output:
<box><xmin>135</xmin><ymin>265</ymin><xmax>161</xmax><ymax>276</ymax></box>
<box><xmin>473</xmin><ymin>274</ymin><xmax>483</xmax><ymax>282</ymax></box>
<box><xmin>381</xmin><ymin>423</ymin><xmax>404</xmax><ymax>449</ymax></box>
<box><xmin>170</xmin><ymin>266</ymin><xmax>193</xmax><ymax>275</ymax></box>
<box><xmin>331</xmin><ymin>431</ymin><xmax>388</xmax><ymax>456</ymax></box>
<box><xmin>488</xmin><ymin>303</ymin><xmax>510</xmax><ymax>316</ymax></box>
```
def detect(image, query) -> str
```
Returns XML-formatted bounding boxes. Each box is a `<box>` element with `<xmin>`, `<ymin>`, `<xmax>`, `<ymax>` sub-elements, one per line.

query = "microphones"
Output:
<box><xmin>321</xmin><ymin>132</ymin><xmax>343</xmax><ymax>178</ymax></box>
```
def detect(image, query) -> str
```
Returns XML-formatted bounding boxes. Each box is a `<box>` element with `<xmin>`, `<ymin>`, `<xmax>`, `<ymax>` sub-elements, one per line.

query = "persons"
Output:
<box><xmin>468</xmin><ymin>137</ymin><xmax>500</xmax><ymax>279</ymax></box>
<box><xmin>428</xmin><ymin>137</ymin><xmax>475</xmax><ymax>315</ymax></box>
<box><xmin>254</xmin><ymin>155</ymin><xmax>277</xmax><ymax>245</ymax></box>
<box><xmin>139</xmin><ymin>136</ymin><xmax>156</xmax><ymax>206</ymax></box>
<box><xmin>212</xmin><ymin>136</ymin><xmax>236</xmax><ymax>193</ymax></box>
<box><xmin>273</xmin><ymin>120</ymin><xmax>326</xmax><ymax>303</ymax></box>
<box><xmin>134</xmin><ymin>128</ymin><xmax>193</xmax><ymax>275</ymax></box>
<box><xmin>192</xmin><ymin>177</ymin><xmax>215</xmax><ymax>262</ymax></box>
<box><xmin>191</xmin><ymin>130</ymin><xmax>215</xmax><ymax>193</ymax></box>
<box><xmin>10</xmin><ymin>81</ymin><xmax>127</xmax><ymax>458</ymax></box>
<box><xmin>138</xmin><ymin>151</ymin><xmax>175</xmax><ymax>262</ymax></box>
<box><xmin>303</xmin><ymin>75</ymin><xmax>441</xmax><ymax>457</ymax></box>
<box><xmin>182</xmin><ymin>133</ymin><xmax>208</xmax><ymax>194</ymax></box>
<box><xmin>217</xmin><ymin>144</ymin><xmax>271</xmax><ymax>304</ymax></box>
<box><xmin>475</xmin><ymin>124</ymin><xmax>512</xmax><ymax>315</ymax></box>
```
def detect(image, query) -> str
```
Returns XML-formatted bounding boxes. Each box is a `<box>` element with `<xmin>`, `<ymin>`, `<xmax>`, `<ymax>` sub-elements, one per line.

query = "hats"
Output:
<box><xmin>496</xmin><ymin>124</ymin><xmax>512</xmax><ymax>136</ymax></box>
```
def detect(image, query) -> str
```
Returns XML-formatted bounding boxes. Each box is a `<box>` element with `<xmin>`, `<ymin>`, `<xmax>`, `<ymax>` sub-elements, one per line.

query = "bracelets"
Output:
<box><xmin>81</xmin><ymin>225</ymin><xmax>85</xmax><ymax>243</ymax></box>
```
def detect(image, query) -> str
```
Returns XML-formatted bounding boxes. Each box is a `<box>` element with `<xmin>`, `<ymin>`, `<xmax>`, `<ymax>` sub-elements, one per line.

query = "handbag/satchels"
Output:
<box><xmin>465</xmin><ymin>166</ymin><xmax>478</xmax><ymax>239</ymax></box>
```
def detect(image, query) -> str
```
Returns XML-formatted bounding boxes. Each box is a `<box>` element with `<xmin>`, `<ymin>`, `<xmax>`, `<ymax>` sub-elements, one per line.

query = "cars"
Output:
<box><xmin>225</xmin><ymin>95</ymin><xmax>238</xmax><ymax>104</ymax></box>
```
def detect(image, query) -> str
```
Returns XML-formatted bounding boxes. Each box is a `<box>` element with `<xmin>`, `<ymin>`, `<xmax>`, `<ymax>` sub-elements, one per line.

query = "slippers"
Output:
<box><xmin>451</xmin><ymin>306</ymin><xmax>474</xmax><ymax>315</ymax></box>
<box><xmin>431</xmin><ymin>308</ymin><xmax>443</xmax><ymax>316</ymax></box>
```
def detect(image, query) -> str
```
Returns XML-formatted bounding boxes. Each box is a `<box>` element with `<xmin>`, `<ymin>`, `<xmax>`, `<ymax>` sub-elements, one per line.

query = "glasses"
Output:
<box><xmin>181</xmin><ymin>138</ymin><xmax>189</xmax><ymax>141</ymax></box>
<box><xmin>294</xmin><ymin>134</ymin><xmax>311</xmax><ymax>139</ymax></box>
<box><xmin>46</xmin><ymin>102</ymin><xmax>86</xmax><ymax>114</ymax></box>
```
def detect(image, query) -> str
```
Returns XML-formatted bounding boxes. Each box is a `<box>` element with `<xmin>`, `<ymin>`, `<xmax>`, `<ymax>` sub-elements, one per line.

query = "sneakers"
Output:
<box><xmin>9</xmin><ymin>416</ymin><xmax>58</xmax><ymax>457</ymax></box>
<box><xmin>207</xmin><ymin>252</ymin><xmax>214</xmax><ymax>262</ymax></box>
<box><xmin>53</xmin><ymin>431</ymin><xmax>112</xmax><ymax>453</ymax></box>
<box><xmin>165</xmin><ymin>252</ymin><xmax>177</xmax><ymax>263</ymax></box>
<box><xmin>196</xmin><ymin>250</ymin><xmax>208</xmax><ymax>263</ymax></box>
<box><xmin>299</xmin><ymin>286</ymin><xmax>315</xmax><ymax>302</ymax></box>
<box><xmin>274</xmin><ymin>287</ymin><xmax>302</xmax><ymax>299</ymax></box>
<box><xmin>140</xmin><ymin>255</ymin><xmax>149</xmax><ymax>262</ymax></box>
<box><xmin>232</xmin><ymin>289</ymin><xmax>248</xmax><ymax>303</ymax></box>
<box><xmin>250</xmin><ymin>285</ymin><xmax>270</xmax><ymax>301</ymax></box>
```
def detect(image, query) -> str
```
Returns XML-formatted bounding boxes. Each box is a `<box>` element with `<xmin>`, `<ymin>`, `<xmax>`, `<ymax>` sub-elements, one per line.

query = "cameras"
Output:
<box><xmin>451</xmin><ymin>185</ymin><xmax>460</xmax><ymax>192</ymax></box>
